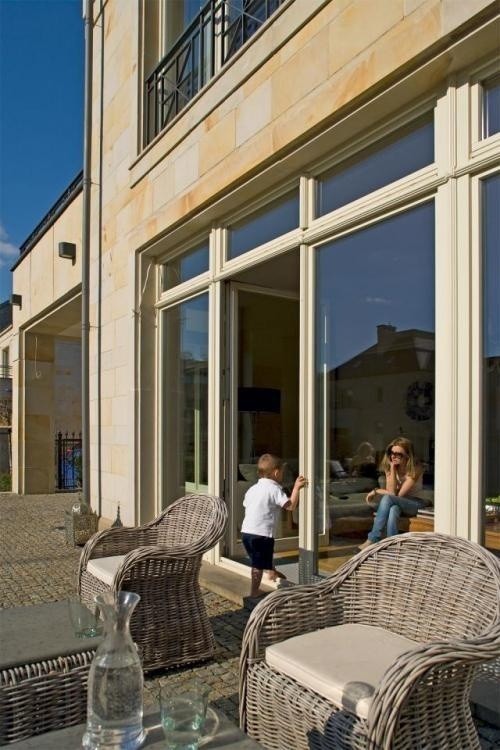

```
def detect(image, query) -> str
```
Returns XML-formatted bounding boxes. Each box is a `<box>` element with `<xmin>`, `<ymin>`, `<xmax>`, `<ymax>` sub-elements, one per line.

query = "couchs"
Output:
<box><xmin>236</xmin><ymin>480</ymin><xmax>410</xmax><ymax>532</ymax></box>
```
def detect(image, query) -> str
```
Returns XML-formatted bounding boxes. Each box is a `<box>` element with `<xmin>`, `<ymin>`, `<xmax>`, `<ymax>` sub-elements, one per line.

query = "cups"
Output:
<box><xmin>64</xmin><ymin>583</ymin><xmax>105</xmax><ymax>639</ymax></box>
<box><xmin>156</xmin><ymin>678</ymin><xmax>214</xmax><ymax>749</ymax></box>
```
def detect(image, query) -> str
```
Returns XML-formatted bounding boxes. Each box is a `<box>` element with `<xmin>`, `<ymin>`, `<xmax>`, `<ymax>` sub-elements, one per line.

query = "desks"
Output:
<box><xmin>0</xmin><ymin>598</ymin><xmax>145</xmax><ymax>744</ymax></box>
<box><xmin>410</xmin><ymin>517</ymin><xmax>499</xmax><ymax>550</ymax></box>
<box><xmin>3</xmin><ymin>700</ymin><xmax>268</xmax><ymax>750</ymax></box>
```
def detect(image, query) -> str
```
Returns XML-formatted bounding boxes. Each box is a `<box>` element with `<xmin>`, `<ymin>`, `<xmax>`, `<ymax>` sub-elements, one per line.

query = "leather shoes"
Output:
<box><xmin>352</xmin><ymin>546</ymin><xmax>362</xmax><ymax>555</ymax></box>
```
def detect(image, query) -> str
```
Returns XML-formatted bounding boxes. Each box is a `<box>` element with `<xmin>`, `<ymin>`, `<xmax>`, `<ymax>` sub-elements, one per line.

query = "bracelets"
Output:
<box><xmin>374</xmin><ymin>487</ymin><xmax>377</xmax><ymax>496</ymax></box>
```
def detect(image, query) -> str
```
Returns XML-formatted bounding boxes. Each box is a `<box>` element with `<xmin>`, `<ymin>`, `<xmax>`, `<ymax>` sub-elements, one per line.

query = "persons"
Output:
<box><xmin>357</xmin><ymin>437</ymin><xmax>424</xmax><ymax>551</ymax></box>
<box><xmin>240</xmin><ymin>454</ymin><xmax>306</xmax><ymax>599</ymax></box>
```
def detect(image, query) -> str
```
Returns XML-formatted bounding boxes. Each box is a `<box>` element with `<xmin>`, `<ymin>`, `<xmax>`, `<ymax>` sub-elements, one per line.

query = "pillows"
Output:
<box><xmin>239</xmin><ymin>463</ymin><xmax>295</xmax><ymax>487</ymax></box>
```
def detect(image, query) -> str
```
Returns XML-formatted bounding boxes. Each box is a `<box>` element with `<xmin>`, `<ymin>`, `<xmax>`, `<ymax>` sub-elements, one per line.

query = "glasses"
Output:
<box><xmin>390</xmin><ymin>451</ymin><xmax>408</xmax><ymax>461</ymax></box>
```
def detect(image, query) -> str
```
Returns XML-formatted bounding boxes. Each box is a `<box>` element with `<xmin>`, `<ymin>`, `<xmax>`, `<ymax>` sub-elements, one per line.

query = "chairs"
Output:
<box><xmin>239</xmin><ymin>530</ymin><xmax>500</xmax><ymax>749</ymax></box>
<box><xmin>78</xmin><ymin>494</ymin><xmax>229</xmax><ymax>674</ymax></box>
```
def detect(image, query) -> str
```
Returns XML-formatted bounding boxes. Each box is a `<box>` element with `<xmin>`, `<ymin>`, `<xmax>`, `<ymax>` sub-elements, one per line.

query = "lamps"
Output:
<box><xmin>237</xmin><ymin>387</ymin><xmax>281</xmax><ymax>461</ymax></box>
